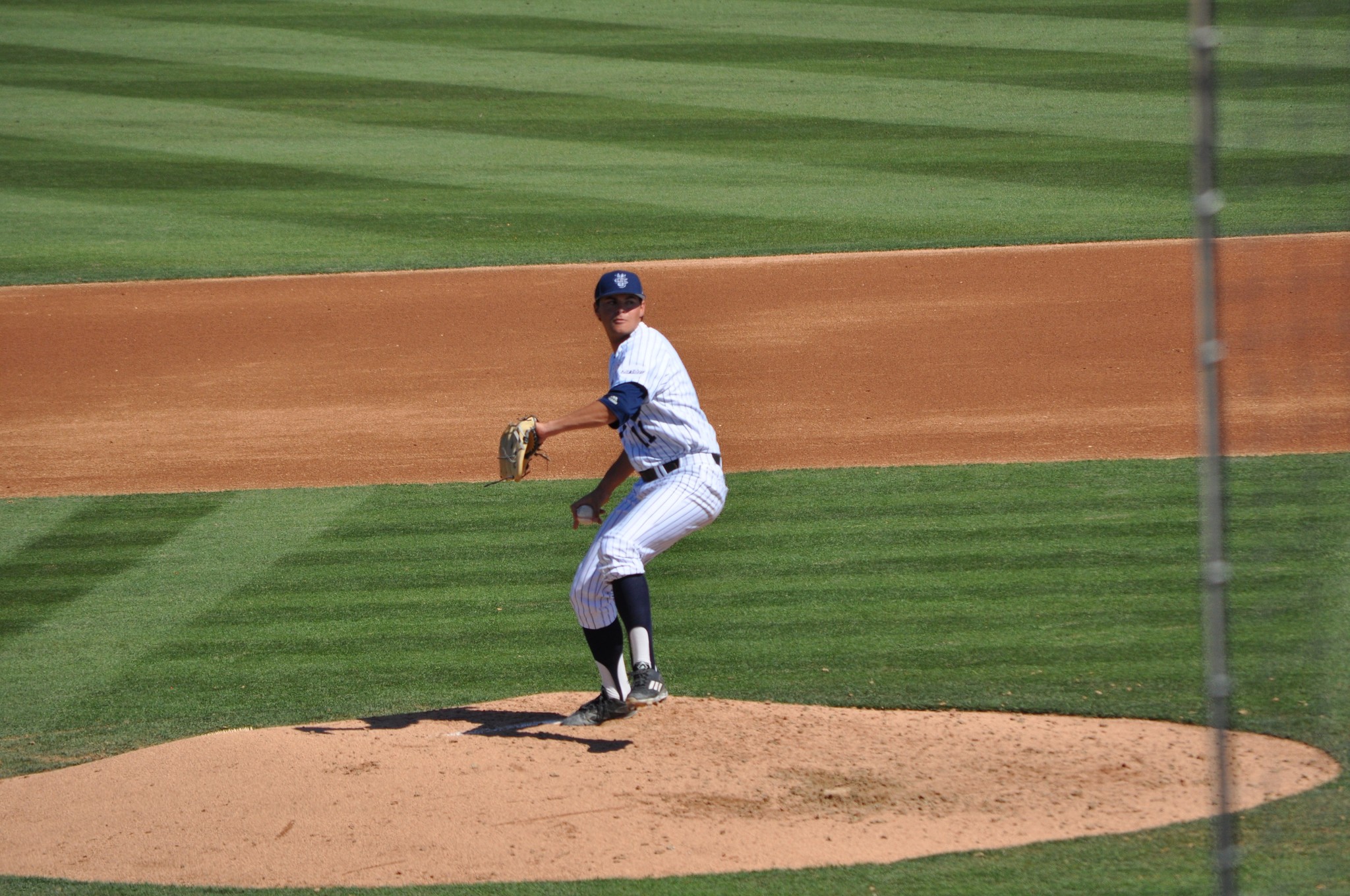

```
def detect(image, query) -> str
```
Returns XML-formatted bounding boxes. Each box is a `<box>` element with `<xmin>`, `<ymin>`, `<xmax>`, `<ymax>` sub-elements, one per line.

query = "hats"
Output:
<box><xmin>595</xmin><ymin>270</ymin><xmax>646</xmax><ymax>302</ymax></box>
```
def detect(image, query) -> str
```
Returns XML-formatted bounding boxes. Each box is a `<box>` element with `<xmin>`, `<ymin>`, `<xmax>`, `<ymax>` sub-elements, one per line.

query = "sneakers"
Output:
<box><xmin>625</xmin><ymin>669</ymin><xmax>669</xmax><ymax>709</ymax></box>
<box><xmin>560</xmin><ymin>685</ymin><xmax>638</xmax><ymax>726</ymax></box>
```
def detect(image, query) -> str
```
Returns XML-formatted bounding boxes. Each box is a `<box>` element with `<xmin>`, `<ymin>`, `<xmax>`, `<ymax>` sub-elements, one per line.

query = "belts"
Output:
<box><xmin>640</xmin><ymin>454</ymin><xmax>719</xmax><ymax>483</ymax></box>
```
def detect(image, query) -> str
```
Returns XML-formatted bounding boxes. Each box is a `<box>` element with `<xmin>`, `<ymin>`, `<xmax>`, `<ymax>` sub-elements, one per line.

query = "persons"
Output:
<box><xmin>524</xmin><ymin>270</ymin><xmax>729</xmax><ymax>727</ymax></box>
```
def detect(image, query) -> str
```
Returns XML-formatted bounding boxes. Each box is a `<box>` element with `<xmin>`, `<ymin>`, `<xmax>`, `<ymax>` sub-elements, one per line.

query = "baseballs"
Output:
<box><xmin>577</xmin><ymin>506</ymin><xmax>595</xmax><ymax>525</ymax></box>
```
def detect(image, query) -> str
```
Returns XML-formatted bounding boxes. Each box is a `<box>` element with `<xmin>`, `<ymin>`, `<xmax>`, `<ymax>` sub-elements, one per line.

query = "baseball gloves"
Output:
<box><xmin>498</xmin><ymin>415</ymin><xmax>541</xmax><ymax>481</ymax></box>
